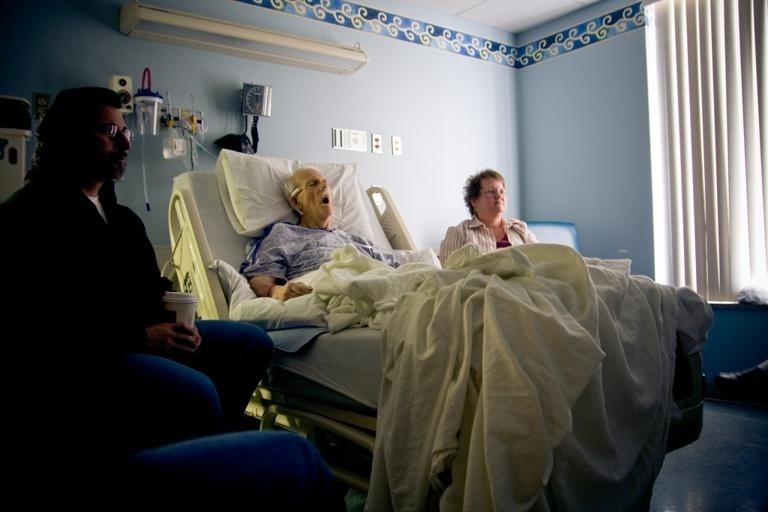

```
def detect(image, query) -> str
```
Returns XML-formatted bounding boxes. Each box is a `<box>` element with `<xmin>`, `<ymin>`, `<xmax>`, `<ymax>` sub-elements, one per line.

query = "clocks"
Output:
<box><xmin>241</xmin><ymin>81</ymin><xmax>272</xmax><ymax>119</ymax></box>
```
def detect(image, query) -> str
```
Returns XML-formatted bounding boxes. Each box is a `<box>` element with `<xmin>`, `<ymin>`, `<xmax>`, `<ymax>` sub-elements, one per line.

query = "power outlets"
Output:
<box><xmin>332</xmin><ymin>127</ymin><xmax>401</xmax><ymax>156</ymax></box>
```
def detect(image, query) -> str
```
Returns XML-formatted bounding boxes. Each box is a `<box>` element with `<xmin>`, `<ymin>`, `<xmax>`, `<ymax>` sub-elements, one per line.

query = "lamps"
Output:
<box><xmin>118</xmin><ymin>3</ymin><xmax>370</xmax><ymax>75</ymax></box>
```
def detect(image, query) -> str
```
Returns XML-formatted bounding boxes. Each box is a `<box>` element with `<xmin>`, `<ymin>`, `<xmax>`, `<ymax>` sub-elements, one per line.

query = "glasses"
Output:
<box><xmin>82</xmin><ymin>121</ymin><xmax>133</xmax><ymax>143</ymax></box>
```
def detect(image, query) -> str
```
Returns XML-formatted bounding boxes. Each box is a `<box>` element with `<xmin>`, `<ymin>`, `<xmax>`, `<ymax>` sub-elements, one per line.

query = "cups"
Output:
<box><xmin>160</xmin><ymin>289</ymin><xmax>197</xmax><ymax>332</ymax></box>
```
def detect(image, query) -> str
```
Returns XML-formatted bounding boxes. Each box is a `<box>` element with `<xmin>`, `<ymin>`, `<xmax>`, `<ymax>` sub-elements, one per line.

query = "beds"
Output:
<box><xmin>167</xmin><ymin>149</ymin><xmax>714</xmax><ymax>512</ymax></box>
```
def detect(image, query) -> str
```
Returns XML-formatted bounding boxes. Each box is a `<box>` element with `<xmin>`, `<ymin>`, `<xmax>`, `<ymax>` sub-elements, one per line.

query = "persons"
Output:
<box><xmin>714</xmin><ymin>358</ymin><xmax>768</xmax><ymax>395</ymax></box>
<box><xmin>0</xmin><ymin>86</ymin><xmax>351</xmax><ymax>451</ymax></box>
<box><xmin>0</xmin><ymin>427</ymin><xmax>349</xmax><ymax>512</ymax></box>
<box><xmin>435</xmin><ymin>169</ymin><xmax>543</xmax><ymax>266</ymax></box>
<box><xmin>240</xmin><ymin>165</ymin><xmax>403</xmax><ymax>300</ymax></box>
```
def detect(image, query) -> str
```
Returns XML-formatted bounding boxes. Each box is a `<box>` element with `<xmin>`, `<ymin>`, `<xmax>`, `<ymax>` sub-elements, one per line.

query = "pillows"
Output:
<box><xmin>213</xmin><ymin>149</ymin><xmax>378</xmax><ymax>248</ymax></box>
